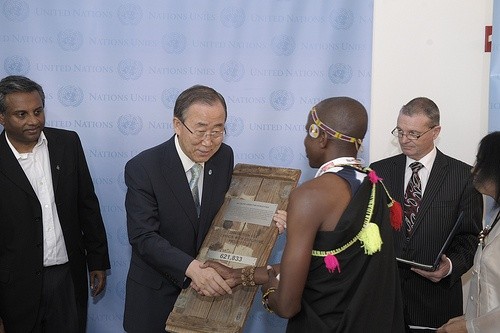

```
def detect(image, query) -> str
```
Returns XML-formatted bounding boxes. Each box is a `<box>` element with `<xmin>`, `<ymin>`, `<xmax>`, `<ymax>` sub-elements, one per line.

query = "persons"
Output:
<box><xmin>368</xmin><ymin>97</ymin><xmax>485</xmax><ymax>333</ymax></box>
<box><xmin>0</xmin><ymin>74</ymin><xmax>111</xmax><ymax>333</ymax></box>
<box><xmin>434</xmin><ymin>131</ymin><xmax>500</xmax><ymax>333</ymax></box>
<box><xmin>122</xmin><ymin>83</ymin><xmax>233</xmax><ymax>333</ymax></box>
<box><xmin>189</xmin><ymin>94</ymin><xmax>407</xmax><ymax>333</ymax></box>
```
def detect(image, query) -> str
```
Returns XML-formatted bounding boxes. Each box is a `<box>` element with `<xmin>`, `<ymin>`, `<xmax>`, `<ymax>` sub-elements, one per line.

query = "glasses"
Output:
<box><xmin>391</xmin><ymin>125</ymin><xmax>437</xmax><ymax>140</ymax></box>
<box><xmin>180</xmin><ymin>119</ymin><xmax>227</xmax><ymax>142</ymax></box>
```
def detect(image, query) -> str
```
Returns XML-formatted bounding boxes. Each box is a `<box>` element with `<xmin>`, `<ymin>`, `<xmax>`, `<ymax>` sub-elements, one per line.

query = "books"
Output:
<box><xmin>394</xmin><ymin>211</ymin><xmax>466</xmax><ymax>272</ymax></box>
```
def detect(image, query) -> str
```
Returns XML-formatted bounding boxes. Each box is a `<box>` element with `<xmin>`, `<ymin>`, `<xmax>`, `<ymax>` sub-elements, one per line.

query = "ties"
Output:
<box><xmin>404</xmin><ymin>162</ymin><xmax>425</xmax><ymax>242</ymax></box>
<box><xmin>189</xmin><ymin>164</ymin><xmax>203</xmax><ymax>219</ymax></box>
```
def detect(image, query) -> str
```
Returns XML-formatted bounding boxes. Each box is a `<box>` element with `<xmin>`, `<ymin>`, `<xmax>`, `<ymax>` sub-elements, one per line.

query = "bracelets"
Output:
<box><xmin>241</xmin><ymin>265</ymin><xmax>256</xmax><ymax>287</ymax></box>
<box><xmin>261</xmin><ymin>287</ymin><xmax>277</xmax><ymax>313</ymax></box>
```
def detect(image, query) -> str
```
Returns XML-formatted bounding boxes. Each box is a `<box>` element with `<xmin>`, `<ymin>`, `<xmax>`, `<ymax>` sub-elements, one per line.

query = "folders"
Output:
<box><xmin>396</xmin><ymin>211</ymin><xmax>464</xmax><ymax>271</ymax></box>
